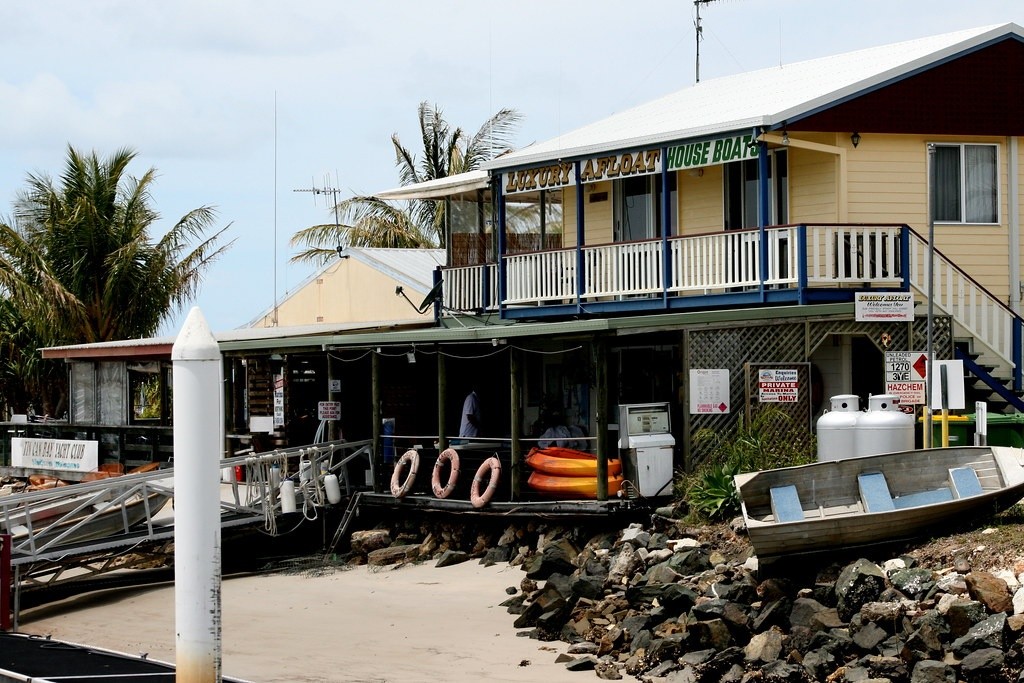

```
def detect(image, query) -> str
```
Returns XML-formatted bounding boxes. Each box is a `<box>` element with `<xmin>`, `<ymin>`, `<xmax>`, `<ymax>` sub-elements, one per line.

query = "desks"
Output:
<box><xmin>414</xmin><ymin>442</ymin><xmax>502</xmax><ymax>451</ymax></box>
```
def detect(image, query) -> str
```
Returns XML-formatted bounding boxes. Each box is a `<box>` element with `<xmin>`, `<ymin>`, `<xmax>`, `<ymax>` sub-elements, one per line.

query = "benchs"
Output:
<box><xmin>948</xmin><ymin>467</ymin><xmax>983</xmax><ymax>500</ymax></box>
<box><xmin>770</xmin><ymin>485</ymin><xmax>805</xmax><ymax>523</ymax></box>
<box><xmin>857</xmin><ymin>473</ymin><xmax>896</xmax><ymax>513</ymax></box>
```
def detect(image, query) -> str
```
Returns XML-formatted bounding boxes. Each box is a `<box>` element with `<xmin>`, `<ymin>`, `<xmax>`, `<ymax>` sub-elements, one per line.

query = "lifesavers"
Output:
<box><xmin>468</xmin><ymin>457</ymin><xmax>501</xmax><ymax>507</ymax></box>
<box><xmin>390</xmin><ymin>449</ymin><xmax>420</xmax><ymax>496</ymax></box>
<box><xmin>431</xmin><ymin>449</ymin><xmax>462</xmax><ymax>497</ymax></box>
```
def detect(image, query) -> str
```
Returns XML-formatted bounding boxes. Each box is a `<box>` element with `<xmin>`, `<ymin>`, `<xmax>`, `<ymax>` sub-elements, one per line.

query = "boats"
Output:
<box><xmin>733</xmin><ymin>445</ymin><xmax>1024</xmax><ymax>557</ymax></box>
<box><xmin>6</xmin><ymin>488</ymin><xmax>174</xmax><ymax>550</ymax></box>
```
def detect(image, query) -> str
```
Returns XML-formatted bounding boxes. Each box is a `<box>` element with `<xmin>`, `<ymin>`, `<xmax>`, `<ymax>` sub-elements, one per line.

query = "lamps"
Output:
<box><xmin>336</xmin><ymin>245</ymin><xmax>349</xmax><ymax>259</ymax></box>
<box><xmin>586</xmin><ymin>183</ymin><xmax>599</xmax><ymax>192</ymax></box>
<box><xmin>851</xmin><ymin>131</ymin><xmax>861</xmax><ymax>148</ymax></box>
<box><xmin>782</xmin><ymin>125</ymin><xmax>790</xmax><ymax>145</ymax></box>
<box><xmin>407</xmin><ymin>343</ymin><xmax>418</xmax><ymax>363</ymax></box>
<box><xmin>689</xmin><ymin>168</ymin><xmax>703</xmax><ymax>177</ymax></box>
<box><xmin>269</xmin><ymin>354</ymin><xmax>283</xmax><ymax>360</ymax></box>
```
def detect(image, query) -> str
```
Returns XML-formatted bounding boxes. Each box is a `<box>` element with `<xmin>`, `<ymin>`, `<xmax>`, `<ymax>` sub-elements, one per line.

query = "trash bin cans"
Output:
<box><xmin>919</xmin><ymin>411</ymin><xmax>1024</xmax><ymax>449</ymax></box>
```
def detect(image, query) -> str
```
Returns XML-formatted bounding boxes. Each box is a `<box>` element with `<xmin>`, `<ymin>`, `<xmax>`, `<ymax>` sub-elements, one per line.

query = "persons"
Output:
<box><xmin>458</xmin><ymin>383</ymin><xmax>482</xmax><ymax>438</ymax></box>
